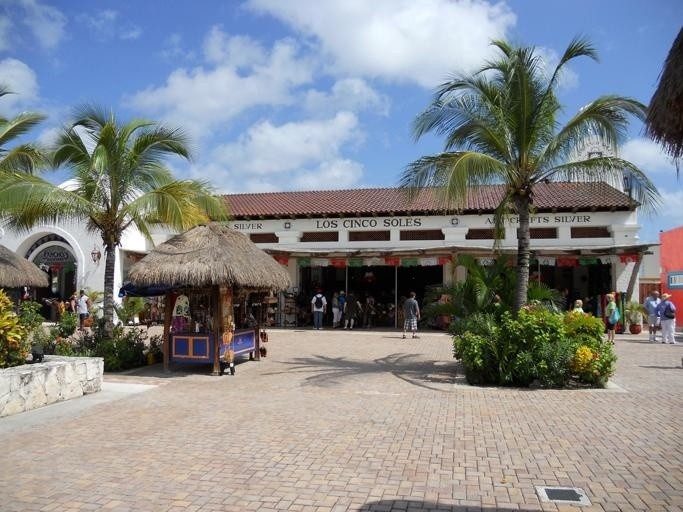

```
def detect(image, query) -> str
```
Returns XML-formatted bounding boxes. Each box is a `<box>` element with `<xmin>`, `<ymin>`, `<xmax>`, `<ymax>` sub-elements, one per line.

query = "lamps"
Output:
<box><xmin>91</xmin><ymin>248</ymin><xmax>101</xmax><ymax>263</ymax></box>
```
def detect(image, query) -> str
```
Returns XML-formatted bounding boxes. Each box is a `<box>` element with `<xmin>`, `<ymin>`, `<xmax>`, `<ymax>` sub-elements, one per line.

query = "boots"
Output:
<box><xmin>332</xmin><ymin>320</ymin><xmax>340</xmax><ymax>328</ymax></box>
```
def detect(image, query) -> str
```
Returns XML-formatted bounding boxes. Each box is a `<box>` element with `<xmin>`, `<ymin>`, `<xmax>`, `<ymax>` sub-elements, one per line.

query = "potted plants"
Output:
<box><xmin>422</xmin><ymin>302</ymin><xmax>460</xmax><ymax>331</ymax></box>
<box><xmin>623</xmin><ymin>300</ymin><xmax>648</xmax><ymax>335</ymax></box>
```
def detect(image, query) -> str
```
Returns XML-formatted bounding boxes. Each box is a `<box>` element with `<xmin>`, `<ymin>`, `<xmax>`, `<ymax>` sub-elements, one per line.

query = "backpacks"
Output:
<box><xmin>314</xmin><ymin>295</ymin><xmax>324</xmax><ymax>308</ymax></box>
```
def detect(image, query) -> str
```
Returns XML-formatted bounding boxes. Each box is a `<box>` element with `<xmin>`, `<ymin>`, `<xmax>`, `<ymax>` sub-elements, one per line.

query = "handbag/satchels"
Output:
<box><xmin>608</xmin><ymin>307</ymin><xmax>620</xmax><ymax>325</ymax></box>
<box><xmin>664</xmin><ymin>303</ymin><xmax>675</xmax><ymax>319</ymax></box>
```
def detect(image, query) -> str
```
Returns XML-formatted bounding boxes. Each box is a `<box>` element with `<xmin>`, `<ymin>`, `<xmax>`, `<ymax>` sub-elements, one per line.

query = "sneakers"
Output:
<box><xmin>402</xmin><ymin>334</ymin><xmax>419</xmax><ymax>339</ymax></box>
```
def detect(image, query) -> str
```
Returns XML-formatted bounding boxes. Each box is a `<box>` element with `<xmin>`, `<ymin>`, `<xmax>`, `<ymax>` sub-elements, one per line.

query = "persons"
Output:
<box><xmin>331</xmin><ymin>290</ymin><xmax>377</xmax><ymax>329</ymax></box>
<box><xmin>653</xmin><ymin>292</ymin><xmax>678</xmax><ymax>344</ymax></box>
<box><xmin>402</xmin><ymin>291</ymin><xmax>420</xmax><ymax>339</ymax></box>
<box><xmin>493</xmin><ymin>293</ymin><xmax>500</xmax><ymax>306</ymax></box>
<box><xmin>139</xmin><ymin>303</ymin><xmax>151</xmax><ymax>325</ymax></box>
<box><xmin>604</xmin><ymin>293</ymin><xmax>617</xmax><ymax>345</ymax></box>
<box><xmin>20</xmin><ymin>286</ymin><xmax>29</xmax><ymax>301</ymax></box>
<box><xmin>642</xmin><ymin>290</ymin><xmax>662</xmax><ymax>343</ymax></box>
<box><xmin>42</xmin><ymin>289</ymin><xmax>91</xmax><ymax>331</ymax></box>
<box><xmin>311</xmin><ymin>288</ymin><xmax>327</xmax><ymax>330</ymax></box>
<box><xmin>151</xmin><ymin>304</ymin><xmax>161</xmax><ymax>322</ymax></box>
<box><xmin>560</xmin><ymin>287</ymin><xmax>597</xmax><ymax>317</ymax></box>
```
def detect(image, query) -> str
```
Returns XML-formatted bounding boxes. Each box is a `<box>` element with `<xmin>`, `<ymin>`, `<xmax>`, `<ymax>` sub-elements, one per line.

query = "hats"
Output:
<box><xmin>661</xmin><ymin>293</ymin><xmax>672</xmax><ymax>301</ymax></box>
<box><xmin>316</xmin><ymin>288</ymin><xmax>323</xmax><ymax>293</ymax></box>
